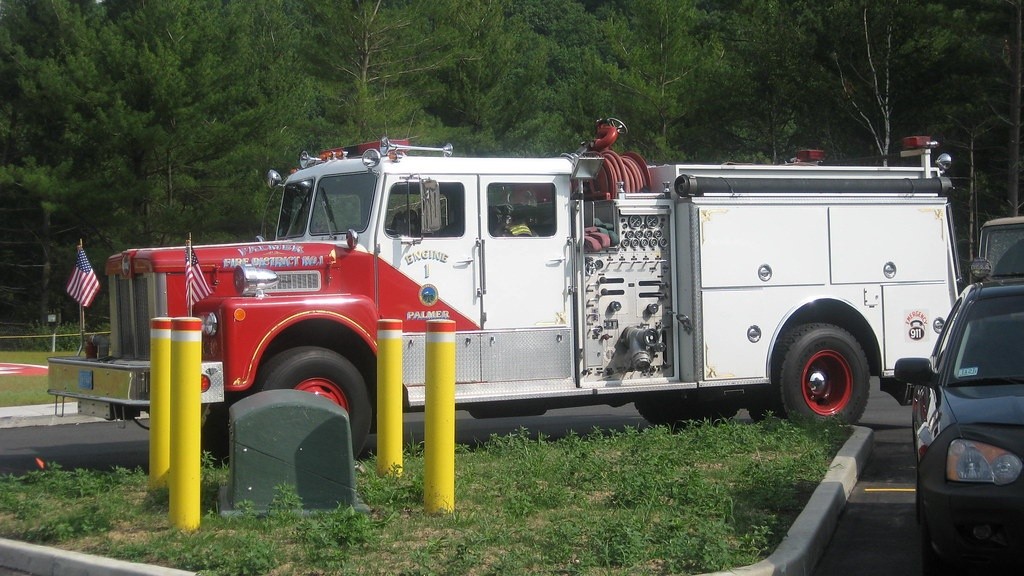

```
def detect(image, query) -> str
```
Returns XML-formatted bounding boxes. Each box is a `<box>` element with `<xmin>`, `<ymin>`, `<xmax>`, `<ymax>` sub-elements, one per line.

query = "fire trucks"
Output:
<box><xmin>47</xmin><ymin>111</ymin><xmax>962</xmax><ymax>458</ymax></box>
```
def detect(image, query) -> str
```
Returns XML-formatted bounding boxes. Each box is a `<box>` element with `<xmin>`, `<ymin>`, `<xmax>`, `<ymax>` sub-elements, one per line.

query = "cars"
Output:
<box><xmin>971</xmin><ymin>216</ymin><xmax>1024</xmax><ymax>284</ymax></box>
<box><xmin>894</xmin><ymin>282</ymin><xmax>1024</xmax><ymax>576</ymax></box>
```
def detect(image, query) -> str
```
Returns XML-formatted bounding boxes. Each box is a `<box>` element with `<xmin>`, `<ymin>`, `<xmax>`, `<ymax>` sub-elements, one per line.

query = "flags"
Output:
<box><xmin>67</xmin><ymin>248</ymin><xmax>101</xmax><ymax>308</ymax></box>
<box><xmin>185</xmin><ymin>246</ymin><xmax>212</xmax><ymax>308</ymax></box>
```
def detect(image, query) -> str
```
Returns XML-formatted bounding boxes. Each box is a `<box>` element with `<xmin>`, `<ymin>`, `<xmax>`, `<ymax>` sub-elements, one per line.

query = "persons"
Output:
<box><xmin>390</xmin><ymin>210</ymin><xmax>420</xmax><ymax>238</ymax></box>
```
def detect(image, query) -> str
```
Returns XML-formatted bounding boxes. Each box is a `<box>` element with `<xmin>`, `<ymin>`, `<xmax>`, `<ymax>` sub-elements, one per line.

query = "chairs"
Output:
<box><xmin>391</xmin><ymin>203</ymin><xmax>540</xmax><ymax>238</ymax></box>
